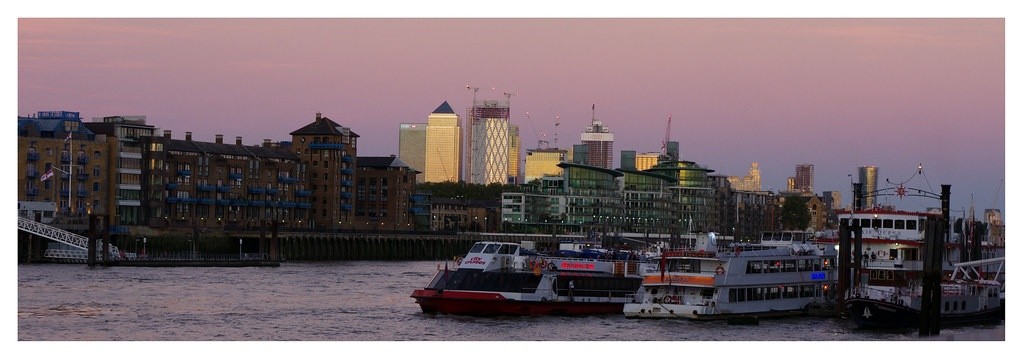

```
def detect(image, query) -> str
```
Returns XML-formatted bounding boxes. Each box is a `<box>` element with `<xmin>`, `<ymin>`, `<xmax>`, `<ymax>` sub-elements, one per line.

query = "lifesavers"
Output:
<box><xmin>716</xmin><ymin>267</ymin><xmax>725</xmax><ymax>276</ymax></box>
<box><xmin>539</xmin><ymin>258</ymin><xmax>547</xmax><ymax>268</ymax></box>
<box><xmin>662</xmin><ymin>295</ymin><xmax>671</xmax><ymax>305</ymax></box>
<box><xmin>547</xmin><ymin>262</ymin><xmax>556</xmax><ymax>271</ymax></box>
<box><xmin>878</xmin><ymin>249</ymin><xmax>885</xmax><ymax>257</ymax></box>
<box><xmin>457</xmin><ymin>257</ymin><xmax>463</xmax><ymax>266</ymax></box>
<box><xmin>529</xmin><ymin>259</ymin><xmax>538</xmax><ymax>270</ymax></box>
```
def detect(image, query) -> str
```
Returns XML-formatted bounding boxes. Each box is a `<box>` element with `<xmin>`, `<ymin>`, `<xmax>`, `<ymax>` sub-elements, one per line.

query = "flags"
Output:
<box><xmin>40</xmin><ymin>170</ymin><xmax>54</xmax><ymax>182</ymax></box>
<box><xmin>64</xmin><ymin>133</ymin><xmax>71</xmax><ymax>141</ymax></box>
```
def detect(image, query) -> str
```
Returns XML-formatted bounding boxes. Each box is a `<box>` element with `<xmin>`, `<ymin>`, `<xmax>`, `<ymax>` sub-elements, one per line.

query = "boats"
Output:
<box><xmin>838</xmin><ymin>202</ymin><xmax>1005</xmax><ymax>305</ymax></box>
<box><xmin>620</xmin><ymin>244</ymin><xmax>843</xmax><ymax>321</ymax></box>
<box><xmin>410</xmin><ymin>241</ymin><xmax>646</xmax><ymax>317</ymax></box>
<box><xmin>842</xmin><ymin>272</ymin><xmax>1001</xmax><ymax>331</ymax></box>
